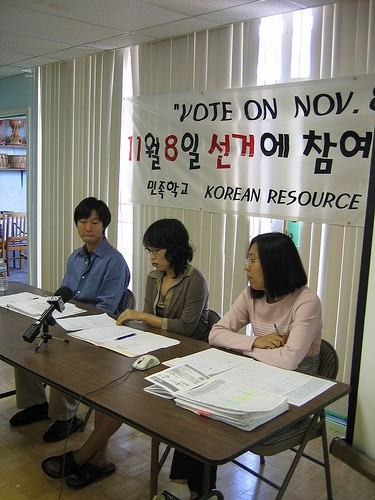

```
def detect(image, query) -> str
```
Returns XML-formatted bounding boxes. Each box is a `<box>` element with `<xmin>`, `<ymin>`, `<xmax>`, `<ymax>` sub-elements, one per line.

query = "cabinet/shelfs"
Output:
<box><xmin>0</xmin><ymin>116</ymin><xmax>26</xmax><ymax>172</ymax></box>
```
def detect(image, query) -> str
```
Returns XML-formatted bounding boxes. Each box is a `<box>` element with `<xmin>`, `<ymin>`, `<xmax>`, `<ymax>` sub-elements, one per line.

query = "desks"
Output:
<box><xmin>0</xmin><ymin>278</ymin><xmax>353</xmax><ymax>500</ymax></box>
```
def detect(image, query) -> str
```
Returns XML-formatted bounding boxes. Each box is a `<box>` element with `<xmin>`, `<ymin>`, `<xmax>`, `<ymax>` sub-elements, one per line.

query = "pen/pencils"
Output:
<box><xmin>33</xmin><ymin>295</ymin><xmax>47</xmax><ymax>300</ymax></box>
<box><xmin>274</xmin><ymin>324</ymin><xmax>281</xmax><ymax>337</ymax></box>
<box><xmin>115</xmin><ymin>332</ymin><xmax>137</xmax><ymax>341</ymax></box>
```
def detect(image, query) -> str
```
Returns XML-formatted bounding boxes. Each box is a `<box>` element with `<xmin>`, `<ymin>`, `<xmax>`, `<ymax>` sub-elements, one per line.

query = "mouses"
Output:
<box><xmin>133</xmin><ymin>355</ymin><xmax>161</xmax><ymax>372</ymax></box>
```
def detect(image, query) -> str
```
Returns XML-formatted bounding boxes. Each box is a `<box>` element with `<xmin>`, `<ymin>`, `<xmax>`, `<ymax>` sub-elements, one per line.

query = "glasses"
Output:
<box><xmin>144</xmin><ymin>246</ymin><xmax>164</xmax><ymax>254</ymax></box>
<box><xmin>245</xmin><ymin>257</ymin><xmax>260</xmax><ymax>265</ymax></box>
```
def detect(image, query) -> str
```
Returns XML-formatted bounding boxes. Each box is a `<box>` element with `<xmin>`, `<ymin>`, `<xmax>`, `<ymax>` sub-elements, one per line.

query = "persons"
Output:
<box><xmin>151</xmin><ymin>232</ymin><xmax>321</xmax><ymax>500</ymax></box>
<box><xmin>41</xmin><ymin>219</ymin><xmax>209</xmax><ymax>488</ymax></box>
<box><xmin>8</xmin><ymin>197</ymin><xmax>130</xmax><ymax>442</ymax></box>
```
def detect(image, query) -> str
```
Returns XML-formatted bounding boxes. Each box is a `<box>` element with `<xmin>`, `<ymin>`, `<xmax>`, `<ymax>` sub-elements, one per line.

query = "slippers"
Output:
<box><xmin>66</xmin><ymin>462</ymin><xmax>116</xmax><ymax>488</ymax></box>
<box><xmin>42</xmin><ymin>450</ymin><xmax>79</xmax><ymax>478</ymax></box>
<box><xmin>153</xmin><ymin>488</ymin><xmax>198</xmax><ymax>500</ymax></box>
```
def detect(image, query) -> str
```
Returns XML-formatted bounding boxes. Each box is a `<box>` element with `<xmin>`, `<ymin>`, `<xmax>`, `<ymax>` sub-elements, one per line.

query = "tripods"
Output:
<box><xmin>33</xmin><ymin>324</ymin><xmax>69</xmax><ymax>351</ymax></box>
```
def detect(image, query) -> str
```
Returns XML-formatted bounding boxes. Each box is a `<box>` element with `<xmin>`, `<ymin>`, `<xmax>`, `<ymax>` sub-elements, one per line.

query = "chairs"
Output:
<box><xmin>78</xmin><ymin>287</ymin><xmax>136</xmax><ymax>433</ymax></box>
<box><xmin>0</xmin><ymin>214</ymin><xmax>5</xmax><ymax>258</ymax></box>
<box><xmin>6</xmin><ymin>213</ymin><xmax>28</xmax><ymax>277</ymax></box>
<box><xmin>208</xmin><ymin>306</ymin><xmax>223</xmax><ymax>330</ymax></box>
<box><xmin>228</xmin><ymin>338</ymin><xmax>339</xmax><ymax>500</ymax></box>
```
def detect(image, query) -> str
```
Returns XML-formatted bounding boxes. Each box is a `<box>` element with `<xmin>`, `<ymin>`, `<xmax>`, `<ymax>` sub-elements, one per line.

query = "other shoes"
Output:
<box><xmin>9</xmin><ymin>402</ymin><xmax>50</xmax><ymax>428</ymax></box>
<box><xmin>43</xmin><ymin>416</ymin><xmax>83</xmax><ymax>442</ymax></box>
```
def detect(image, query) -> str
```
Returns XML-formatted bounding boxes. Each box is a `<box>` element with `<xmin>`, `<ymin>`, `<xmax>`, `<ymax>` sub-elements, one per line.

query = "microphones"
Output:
<box><xmin>22</xmin><ymin>286</ymin><xmax>73</xmax><ymax>343</ymax></box>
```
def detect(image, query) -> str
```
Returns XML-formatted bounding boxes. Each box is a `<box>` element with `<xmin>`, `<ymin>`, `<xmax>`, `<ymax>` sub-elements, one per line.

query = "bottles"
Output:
<box><xmin>0</xmin><ymin>258</ymin><xmax>9</xmax><ymax>292</ymax></box>
